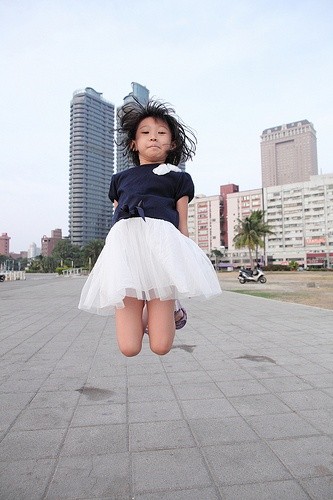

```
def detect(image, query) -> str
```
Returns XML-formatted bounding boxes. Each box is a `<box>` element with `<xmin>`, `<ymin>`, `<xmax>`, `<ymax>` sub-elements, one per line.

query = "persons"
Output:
<box><xmin>77</xmin><ymin>95</ymin><xmax>223</xmax><ymax>357</ymax></box>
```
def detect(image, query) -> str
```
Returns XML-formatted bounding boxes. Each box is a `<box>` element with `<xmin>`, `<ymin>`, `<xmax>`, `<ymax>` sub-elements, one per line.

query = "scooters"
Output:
<box><xmin>237</xmin><ymin>262</ymin><xmax>267</xmax><ymax>284</ymax></box>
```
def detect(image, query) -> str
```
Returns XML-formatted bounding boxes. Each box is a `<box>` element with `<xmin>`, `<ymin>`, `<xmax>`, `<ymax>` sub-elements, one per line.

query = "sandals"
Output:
<box><xmin>173</xmin><ymin>308</ymin><xmax>186</xmax><ymax>330</ymax></box>
